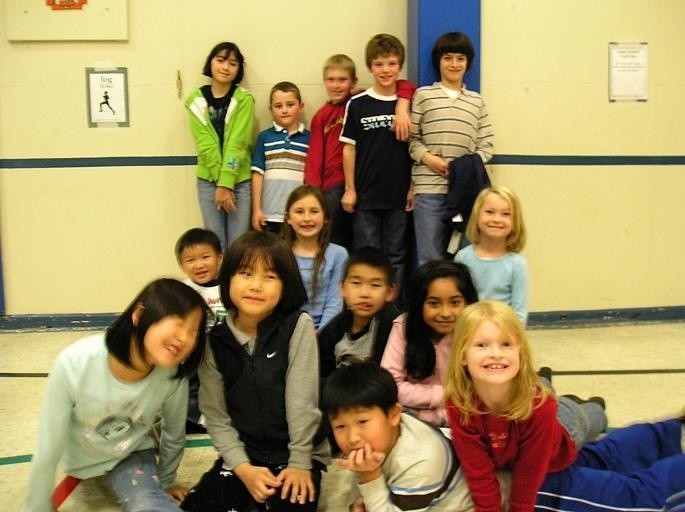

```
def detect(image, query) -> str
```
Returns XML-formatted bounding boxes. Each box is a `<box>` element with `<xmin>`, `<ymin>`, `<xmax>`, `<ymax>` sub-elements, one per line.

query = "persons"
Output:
<box><xmin>25</xmin><ymin>278</ymin><xmax>214</xmax><ymax>512</ymax></box>
<box><xmin>338</xmin><ymin>35</ymin><xmax>416</xmax><ymax>304</ymax></box>
<box><xmin>98</xmin><ymin>91</ymin><xmax>116</xmax><ymax>115</ymax></box>
<box><xmin>250</xmin><ymin>83</ymin><xmax>312</xmax><ymax>232</ymax></box>
<box><xmin>185</xmin><ymin>42</ymin><xmax>254</xmax><ymax>255</ymax></box>
<box><xmin>407</xmin><ymin>33</ymin><xmax>496</xmax><ymax>267</ymax></box>
<box><xmin>305</xmin><ymin>34</ymin><xmax>415</xmax><ymax>247</ymax></box>
<box><xmin>174</xmin><ymin>182</ymin><xmax>685</xmax><ymax>512</ymax></box>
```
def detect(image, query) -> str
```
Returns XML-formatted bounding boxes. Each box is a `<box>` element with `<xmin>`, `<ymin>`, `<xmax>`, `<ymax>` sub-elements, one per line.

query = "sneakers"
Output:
<box><xmin>538</xmin><ymin>367</ymin><xmax>606</xmax><ymax>434</ymax></box>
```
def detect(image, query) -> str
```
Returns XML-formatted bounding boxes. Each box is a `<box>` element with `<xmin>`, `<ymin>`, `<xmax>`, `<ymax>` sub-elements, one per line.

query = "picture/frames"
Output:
<box><xmin>85</xmin><ymin>67</ymin><xmax>130</xmax><ymax>128</ymax></box>
<box><xmin>608</xmin><ymin>42</ymin><xmax>647</xmax><ymax>103</ymax></box>
